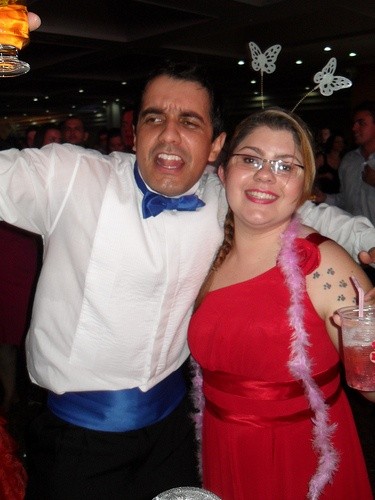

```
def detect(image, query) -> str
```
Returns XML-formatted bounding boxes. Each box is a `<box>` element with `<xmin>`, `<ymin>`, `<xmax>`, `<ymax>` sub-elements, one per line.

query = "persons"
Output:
<box><xmin>186</xmin><ymin>107</ymin><xmax>375</xmax><ymax>500</ymax></box>
<box><xmin>1</xmin><ymin>12</ymin><xmax>375</xmax><ymax>500</ymax></box>
<box><xmin>0</xmin><ymin>104</ymin><xmax>375</xmax><ymax>226</ymax></box>
<box><xmin>0</xmin><ymin>222</ymin><xmax>43</xmax><ymax>421</ymax></box>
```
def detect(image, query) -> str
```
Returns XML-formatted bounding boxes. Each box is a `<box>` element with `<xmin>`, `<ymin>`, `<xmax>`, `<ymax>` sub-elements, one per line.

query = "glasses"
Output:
<box><xmin>227</xmin><ymin>153</ymin><xmax>305</xmax><ymax>177</ymax></box>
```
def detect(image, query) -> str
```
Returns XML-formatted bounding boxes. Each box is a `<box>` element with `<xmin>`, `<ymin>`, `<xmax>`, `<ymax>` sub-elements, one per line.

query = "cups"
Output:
<box><xmin>337</xmin><ymin>304</ymin><xmax>375</xmax><ymax>391</ymax></box>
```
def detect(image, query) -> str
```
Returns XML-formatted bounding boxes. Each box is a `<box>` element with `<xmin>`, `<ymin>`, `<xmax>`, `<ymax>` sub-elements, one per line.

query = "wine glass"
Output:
<box><xmin>0</xmin><ymin>0</ymin><xmax>30</xmax><ymax>78</ymax></box>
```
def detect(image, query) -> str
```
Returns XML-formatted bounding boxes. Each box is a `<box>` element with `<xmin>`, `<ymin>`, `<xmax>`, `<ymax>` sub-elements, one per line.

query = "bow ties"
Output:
<box><xmin>133</xmin><ymin>162</ymin><xmax>206</xmax><ymax>218</ymax></box>
